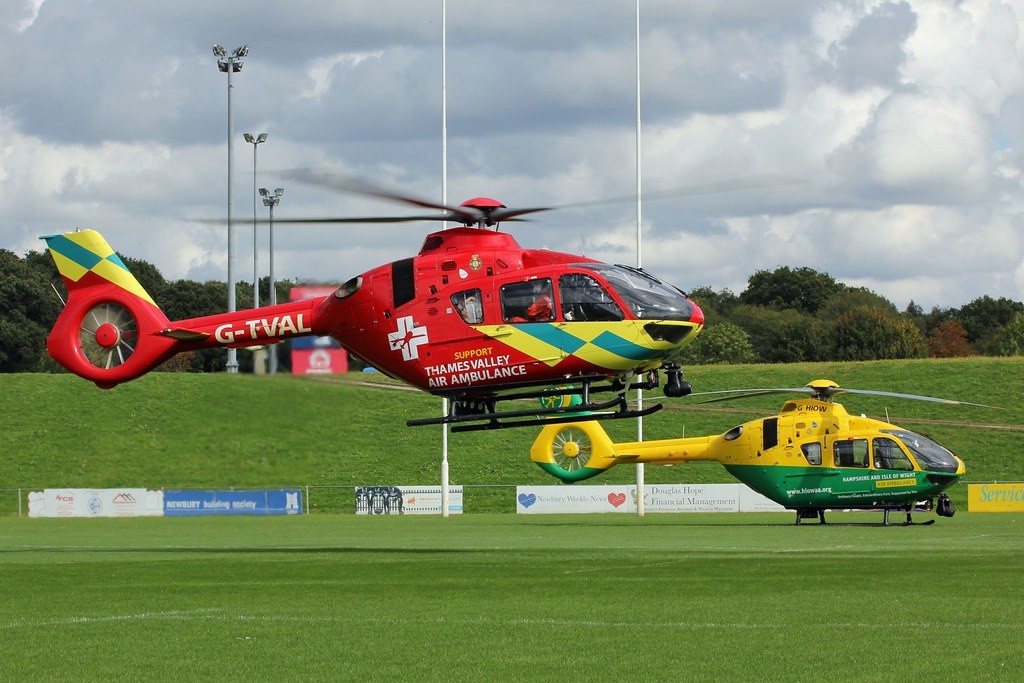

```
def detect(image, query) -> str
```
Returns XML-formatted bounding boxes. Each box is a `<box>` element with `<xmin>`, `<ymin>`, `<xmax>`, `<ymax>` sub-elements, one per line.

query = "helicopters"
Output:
<box><xmin>39</xmin><ymin>153</ymin><xmax>822</xmax><ymax>434</ymax></box>
<box><xmin>530</xmin><ymin>379</ymin><xmax>1008</xmax><ymax>527</ymax></box>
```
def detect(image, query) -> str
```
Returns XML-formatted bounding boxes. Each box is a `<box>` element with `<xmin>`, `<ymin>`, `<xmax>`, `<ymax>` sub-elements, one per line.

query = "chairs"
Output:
<box><xmin>876</xmin><ymin>448</ymin><xmax>892</xmax><ymax>468</ymax></box>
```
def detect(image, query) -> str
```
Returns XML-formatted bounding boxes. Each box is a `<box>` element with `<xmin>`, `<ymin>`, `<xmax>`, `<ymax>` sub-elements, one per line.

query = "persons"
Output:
<box><xmin>509</xmin><ymin>284</ymin><xmax>554</xmax><ymax>322</ymax></box>
<box><xmin>451</xmin><ymin>295</ymin><xmax>478</xmax><ymax>322</ymax></box>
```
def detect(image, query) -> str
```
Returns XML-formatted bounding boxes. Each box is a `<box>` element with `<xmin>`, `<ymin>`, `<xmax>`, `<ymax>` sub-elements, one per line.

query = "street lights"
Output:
<box><xmin>241</xmin><ymin>132</ymin><xmax>265</xmax><ymax>370</ymax></box>
<box><xmin>258</xmin><ymin>188</ymin><xmax>284</xmax><ymax>373</ymax></box>
<box><xmin>212</xmin><ymin>42</ymin><xmax>251</xmax><ymax>371</ymax></box>
